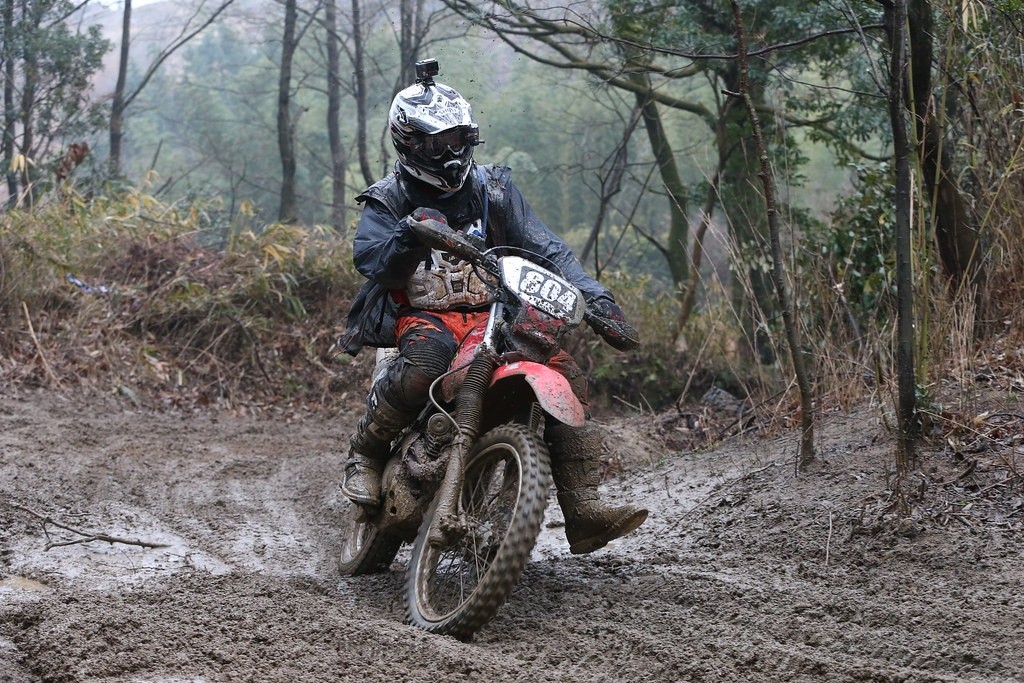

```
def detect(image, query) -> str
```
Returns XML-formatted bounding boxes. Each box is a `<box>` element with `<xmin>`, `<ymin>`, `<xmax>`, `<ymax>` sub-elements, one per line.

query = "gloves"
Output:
<box><xmin>593</xmin><ymin>298</ymin><xmax>625</xmax><ymax>322</ymax></box>
<box><xmin>408</xmin><ymin>208</ymin><xmax>447</xmax><ymax>226</ymax></box>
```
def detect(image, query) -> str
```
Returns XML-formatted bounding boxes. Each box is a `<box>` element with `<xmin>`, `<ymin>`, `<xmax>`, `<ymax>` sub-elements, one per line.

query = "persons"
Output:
<box><xmin>339</xmin><ymin>82</ymin><xmax>649</xmax><ymax>553</ymax></box>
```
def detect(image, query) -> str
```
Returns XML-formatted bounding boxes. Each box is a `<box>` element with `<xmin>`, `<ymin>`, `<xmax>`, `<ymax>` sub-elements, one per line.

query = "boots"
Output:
<box><xmin>341</xmin><ymin>378</ymin><xmax>412</xmax><ymax>504</ymax></box>
<box><xmin>544</xmin><ymin>419</ymin><xmax>649</xmax><ymax>554</ymax></box>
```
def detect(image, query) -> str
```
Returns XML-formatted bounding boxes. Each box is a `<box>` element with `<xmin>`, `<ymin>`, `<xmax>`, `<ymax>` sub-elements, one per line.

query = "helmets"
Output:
<box><xmin>388</xmin><ymin>57</ymin><xmax>478</xmax><ymax>195</ymax></box>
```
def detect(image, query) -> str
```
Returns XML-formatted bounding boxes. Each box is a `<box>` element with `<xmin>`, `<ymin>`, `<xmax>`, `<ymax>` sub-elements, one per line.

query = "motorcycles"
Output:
<box><xmin>338</xmin><ymin>217</ymin><xmax>643</xmax><ymax>640</ymax></box>
